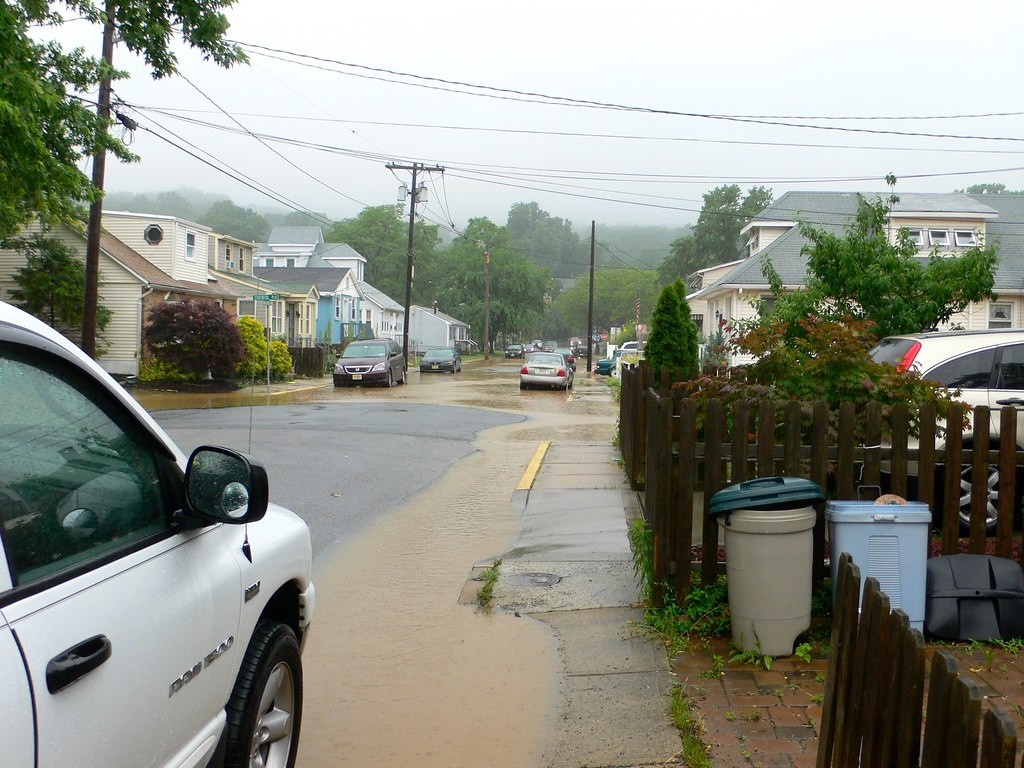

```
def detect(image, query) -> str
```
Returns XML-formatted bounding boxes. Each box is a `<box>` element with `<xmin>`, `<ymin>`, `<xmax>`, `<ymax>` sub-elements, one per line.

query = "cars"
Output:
<box><xmin>524</xmin><ymin>339</ymin><xmax>543</xmax><ymax>353</ymax></box>
<box><xmin>571</xmin><ymin>345</ymin><xmax>588</xmax><ymax>358</ymax></box>
<box><xmin>549</xmin><ymin>347</ymin><xmax>577</xmax><ymax>372</ymax></box>
<box><xmin>504</xmin><ymin>344</ymin><xmax>525</xmax><ymax>359</ymax></box>
<box><xmin>594</xmin><ymin>340</ymin><xmax>648</xmax><ymax>379</ymax></box>
<box><xmin>418</xmin><ymin>348</ymin><xmax>463</xmax><ymax>374</ymax></box>
<box><xmin>519</xmin><ymin>351</ymin><xmax>575</xmax><ymax>390</ymax></box>
<box><xmin>331</xmin><ymin>337</ymin><xmax>408</xmax><ymax>388</ymax></box>
<box><xmin>0</xmin><ymin>300</ymin><xmax>315</xmax><ymax>767</ymax></box>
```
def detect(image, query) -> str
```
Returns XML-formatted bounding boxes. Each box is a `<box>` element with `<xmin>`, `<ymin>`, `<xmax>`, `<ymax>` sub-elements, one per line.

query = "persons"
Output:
<box><xmin>594</xmin><ymin>333</ymin><xmax>601</xmax><ymax>343</ymax></box>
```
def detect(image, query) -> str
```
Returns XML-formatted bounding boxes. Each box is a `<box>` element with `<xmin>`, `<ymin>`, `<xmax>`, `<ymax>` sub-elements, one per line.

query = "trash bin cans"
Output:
<box><xmin>715</xmin><ymin>475</ymin><xmax>823</xmax><ymax>657</ymax></box>
<box><xmin>827</xmin><ymin>496</ymin><xmax>933</xmax><ymax>640</ymax></box>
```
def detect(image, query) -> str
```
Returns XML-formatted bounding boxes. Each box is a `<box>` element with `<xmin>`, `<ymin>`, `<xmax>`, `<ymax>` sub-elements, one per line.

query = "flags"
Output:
<box><xmin>635</xmin><ymin>296</ymin><xmax>640</xmax><ymax>322</ymax></box>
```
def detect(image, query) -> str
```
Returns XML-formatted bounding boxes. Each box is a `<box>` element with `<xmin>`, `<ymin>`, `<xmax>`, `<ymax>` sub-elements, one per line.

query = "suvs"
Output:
<box><xmin>864</xmin><ymin>328</ymin><xmax>1023</xmax><ymax>538</ymax></box>
